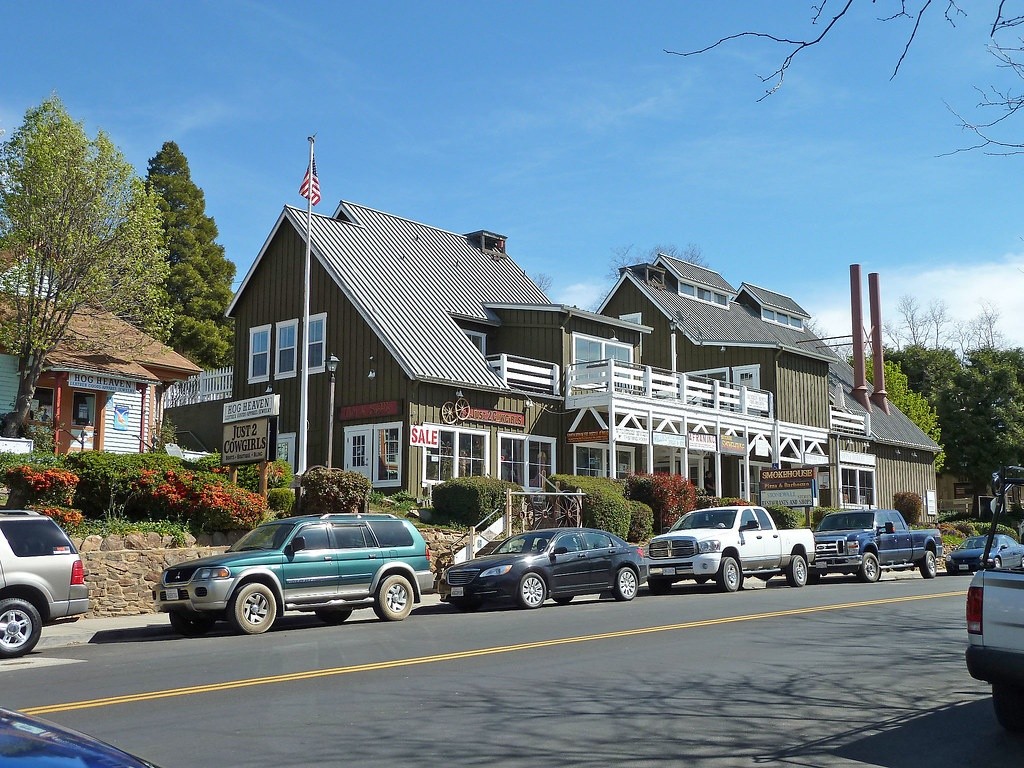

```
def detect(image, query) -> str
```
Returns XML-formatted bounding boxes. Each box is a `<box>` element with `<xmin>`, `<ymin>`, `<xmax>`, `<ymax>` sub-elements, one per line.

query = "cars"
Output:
<box><xmin>436</xmin><ymin>526</ymin><xmax>648</xmax><ymax>611</ymax></box>
<box><xmin>944</xmin><ymin>533</ymin><xmax>1024</xmax><ymax>575</ymax></box>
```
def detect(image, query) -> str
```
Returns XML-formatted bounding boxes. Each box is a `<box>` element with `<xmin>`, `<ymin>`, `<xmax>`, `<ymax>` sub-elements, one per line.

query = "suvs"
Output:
<box><xmin>148</xmin><ymin>511</ymin><xmax>435</xmax><ymax>636</ymax></box>
<box><xmin>0</xmin><ymin>509</ymin><xmax>90</xmax><ymax>659</ymax></box>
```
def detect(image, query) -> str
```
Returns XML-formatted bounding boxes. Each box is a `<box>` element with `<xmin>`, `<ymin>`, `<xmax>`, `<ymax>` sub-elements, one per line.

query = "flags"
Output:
<box><xmin>299</xmin><ymin>152</ymin><xmax>320</xmax><ymax>207</ymax></box>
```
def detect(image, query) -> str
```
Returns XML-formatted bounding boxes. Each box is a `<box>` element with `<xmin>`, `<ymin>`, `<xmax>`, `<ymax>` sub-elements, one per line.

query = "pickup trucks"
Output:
<box><xmin>965</xmin><ymin>564</ymin><xmax>1024</xmax><ymax>734</ymax></box>
<box><xmin>646</xmin><ymin>504</ymin><xmax>816</xmax><ymax>593</ymax></box>
<box><xmin>809</xmin><ymin>508</ymin><xmax>943</xmax><ymax>584</ymax></box>
